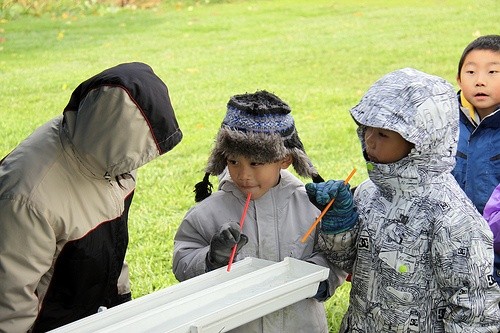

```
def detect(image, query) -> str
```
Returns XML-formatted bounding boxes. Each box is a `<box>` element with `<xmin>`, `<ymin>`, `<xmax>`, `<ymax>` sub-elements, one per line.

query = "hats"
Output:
<box><xmin>193</xmin><ymin>91</ymin><xmax>325</xmax><ymax>202</ymax></box>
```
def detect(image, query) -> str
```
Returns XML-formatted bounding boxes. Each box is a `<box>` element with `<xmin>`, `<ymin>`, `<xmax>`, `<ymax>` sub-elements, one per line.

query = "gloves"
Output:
<box><xmin>305</xmin><ymin>180</ymin><xmax>360</xmax><ymax>235</ymax></box>
<box><xmin>207</xmin><ymin>222</ymin><xmax>247</xmax><ymax>268</ymax></box>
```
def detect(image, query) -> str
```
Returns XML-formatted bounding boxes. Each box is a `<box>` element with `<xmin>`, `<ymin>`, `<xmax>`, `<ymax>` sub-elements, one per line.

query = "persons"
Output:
<box><xmin>305</xmin><ymin>68</ymin><xmax>500</xmax><ymax>333</ymax></box>
<box><xmin>172</xmin><ymin>89</ymin><xmax>350</xmax><ymax>333</ymax></box>
<box><xmin>450</xmin><ymin>34</ymin><xmax>500</xmax><ymax>288</ymax></box>
<box><xmin>0</xmin><ymin>61</ymin><xmax>183</xmax><ymax>333</ymax></box>
